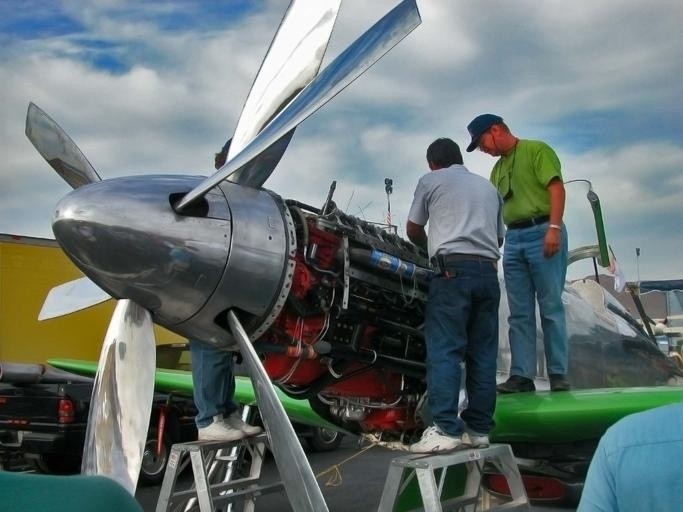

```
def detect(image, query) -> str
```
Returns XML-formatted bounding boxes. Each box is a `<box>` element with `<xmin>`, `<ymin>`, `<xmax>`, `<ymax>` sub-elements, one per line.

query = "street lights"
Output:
<box><xmin>632</xmin><ymin>244</ymin><xmax>639</xmax><ymax>298</ymax></box>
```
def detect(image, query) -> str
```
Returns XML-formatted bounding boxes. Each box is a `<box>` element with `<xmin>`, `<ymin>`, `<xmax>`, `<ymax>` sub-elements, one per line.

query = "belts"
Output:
<box><xmin>436</xmin><ymin>253</ymin><xmax>496</xmax><ymax>264</ymax></box>
<box><xmin>506</xmin><ymin>215</ymin><xmax>548</xmax><ymax>230</ymax></box>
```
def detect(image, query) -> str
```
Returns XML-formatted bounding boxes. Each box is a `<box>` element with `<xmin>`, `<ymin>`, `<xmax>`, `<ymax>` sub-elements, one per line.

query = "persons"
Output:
<box><xmin>405</xmin><ymin>137</ymin><xmax>506</xmax><ymax>454</ymax></box>
<box><xmin>578</xmin><ymin>394</ymin><xmax>682</xmax><ymax>512</ymax></box>
<box><xmin>465</xmin><ymin>113</ymin><xmax>570</xmax><ymax>393</ymax></box>
<box><xmin>190</xmin><ymin>338</ymin><xmax>263</xmax><ymax>444</ymax></box>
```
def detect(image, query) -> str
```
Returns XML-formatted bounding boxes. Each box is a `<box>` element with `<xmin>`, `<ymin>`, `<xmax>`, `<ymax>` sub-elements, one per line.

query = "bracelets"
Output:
<box><xmin>549</xmin><ymin>223</ymin><xmax>563</xmax><ymax>230</ymax></box>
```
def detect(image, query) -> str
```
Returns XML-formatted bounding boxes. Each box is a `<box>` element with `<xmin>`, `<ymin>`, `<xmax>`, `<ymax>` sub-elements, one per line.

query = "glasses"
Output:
<box><xmin>497</xmin><ymin>172</ymin><xmax>513</xmax><ymax>201</ymax></box>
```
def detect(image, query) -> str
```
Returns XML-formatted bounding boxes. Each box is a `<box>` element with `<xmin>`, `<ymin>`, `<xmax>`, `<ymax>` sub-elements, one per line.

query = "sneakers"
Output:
<box><xmin>228</xmin><ymin>410</ymin><xmax>263</xmax><ymax>437</ymax></box>
<box><xmin>407</xmin><ymin>424</ymin><xmax>463</xmax><ymax>455</ymax></box>
<box><xmin>549</xmin><ymin>372</ymin><xmax>574</xmax><ymax>391</ymax></box>
<box><xmin>196</xmin><ymin>414</ymin><xmax>247</xmax><ymax>444</ymax></box>
<box><xmin>461</xmin><ymin>431</ymin><xmax>490</xmax><ymax>449</ymax></box>
<box><xmin>496</xmin><ymin>375</ymin><xmax>537</xmax><ymax>393</ymax></box>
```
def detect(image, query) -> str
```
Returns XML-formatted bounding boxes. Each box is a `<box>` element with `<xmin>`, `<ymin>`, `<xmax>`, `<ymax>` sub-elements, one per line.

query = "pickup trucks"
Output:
<box><xmin>1</xmin><ymin>340</ymin><xmax>348</xmax><ymax>489</ymax></box>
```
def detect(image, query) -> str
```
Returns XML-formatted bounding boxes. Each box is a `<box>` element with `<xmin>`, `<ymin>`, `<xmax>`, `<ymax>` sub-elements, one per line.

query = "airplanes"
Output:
<box><xmin>21</xmin><ymin>0</ymin><xmax>681</xmax><ymax>510</ymax></box>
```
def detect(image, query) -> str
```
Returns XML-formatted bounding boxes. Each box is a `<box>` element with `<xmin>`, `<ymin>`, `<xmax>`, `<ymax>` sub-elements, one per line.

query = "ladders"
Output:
<box><xmin>155</xmin><ymin>431</ymin><xmax>285</xmax><ymax>512</ymax></box>
<box><xmin>377</xmin><ymin>443</ymin><xmax>531</xmax><ymax>512</ymax></box>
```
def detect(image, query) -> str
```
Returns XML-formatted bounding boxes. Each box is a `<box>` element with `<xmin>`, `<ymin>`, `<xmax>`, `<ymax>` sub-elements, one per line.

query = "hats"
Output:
<box><xmin>465</xmin><ymin>113</ymin><xmax>502</xmax><ymax>152</ymax></box>
